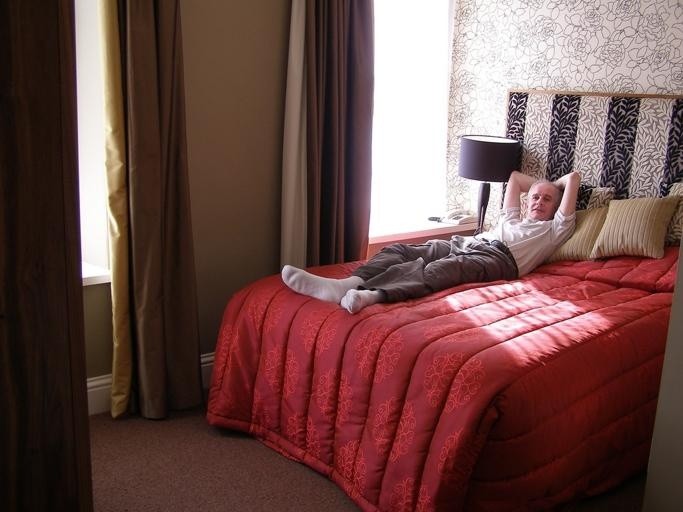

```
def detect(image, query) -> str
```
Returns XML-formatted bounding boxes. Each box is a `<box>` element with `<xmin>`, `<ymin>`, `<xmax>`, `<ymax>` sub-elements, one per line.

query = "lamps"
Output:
<box><xmin>456</xmin><ymin>133</ymin><xmax>521</xmax><ymax>233</ymax></box>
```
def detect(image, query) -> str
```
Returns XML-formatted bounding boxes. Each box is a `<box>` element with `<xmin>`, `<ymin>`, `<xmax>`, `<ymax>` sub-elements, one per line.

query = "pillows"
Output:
<box><xmin>654</xmin><ymin>179</ymin><xmax>681</xmax><ymax>243</ymax></box>
<box><xmin>589</xmin><ymin>195</ymin><xmax>680</xmax><ymax>262</ymax></box>
<box><xmin>573</xmin><ymin>186</ymin><xmax>613</xmax><ymax>208</ymax></box>
<box><xmin>547</xmin><ymin>201</ymin><xmax>600</xmax><ymax>264</ymax></box>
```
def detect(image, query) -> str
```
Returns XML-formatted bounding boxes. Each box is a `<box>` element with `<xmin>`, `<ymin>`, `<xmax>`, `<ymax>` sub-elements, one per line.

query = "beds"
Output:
<box><xmin>205</xmin><ymin>88</ymin><xmax>682</xmax><ymax>511</ymax></box>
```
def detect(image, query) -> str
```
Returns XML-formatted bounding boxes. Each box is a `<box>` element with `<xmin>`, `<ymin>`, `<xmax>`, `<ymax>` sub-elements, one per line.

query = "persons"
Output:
<box><xmin>281</xmin><ymin>171</ymin><xmax>582</xmax><ymax>314</ymax></box>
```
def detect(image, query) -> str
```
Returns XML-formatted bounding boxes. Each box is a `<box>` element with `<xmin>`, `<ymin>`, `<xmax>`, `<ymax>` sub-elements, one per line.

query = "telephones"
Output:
<box><xmin>441</xmin><ymin>209</ymin><xmax>477</xmax><ymax>225</ymax></box>
